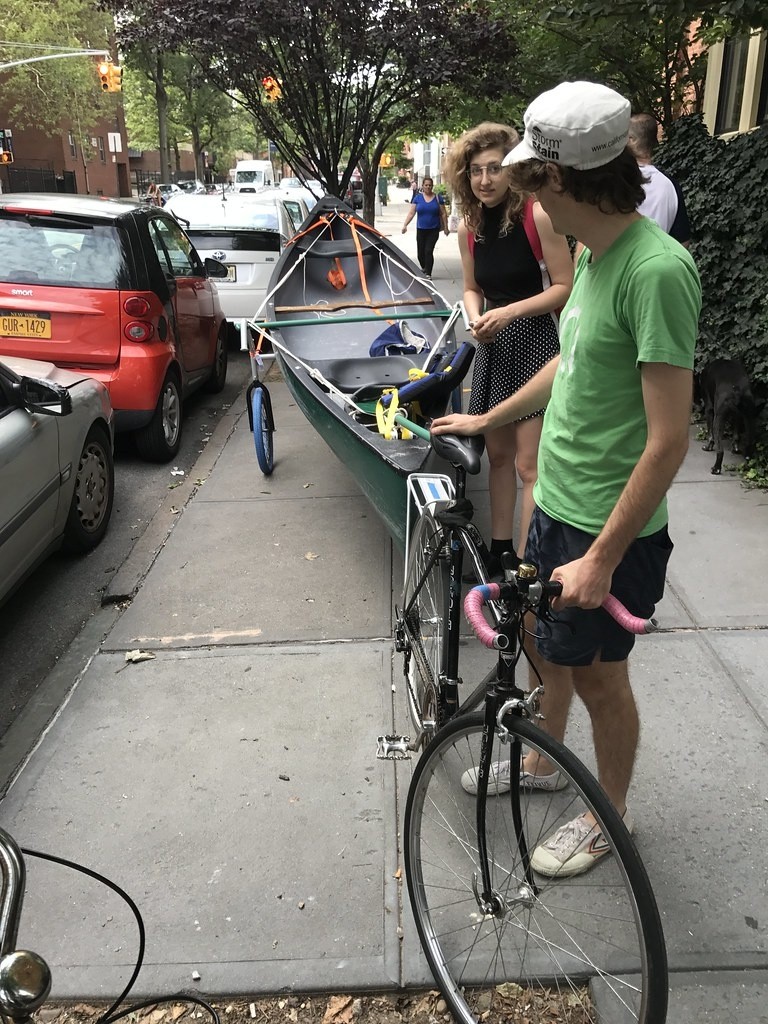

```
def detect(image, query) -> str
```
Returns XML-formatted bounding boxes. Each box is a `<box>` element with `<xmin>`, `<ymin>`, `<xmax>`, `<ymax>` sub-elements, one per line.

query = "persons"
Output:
<box><xmin>403</xmin><ymin>177</ymin><xmax>451</xmax><ymax>280</ymax></box>
<box><xmin>444</xmin><ymin>121</ymin><xmax>575</xmax><ymax>588</ymax></box>
<box><xmin>146</xmin><ymin>180</ymin><xmax>163</xmax><ymax>208</ymax></box>
<box><xmin>408</xmin><ymin>179</ymin><xmax>418</xmax><ymax>204</ymax></box>
<box><xmin>574</xmin><ymin>113</ymin><xmax>678</xmax><ymax>264</ymax></box>
<box><xmin>427</xmin><ymin>80</ymin><xmax>702</xmax><ymax>878</ymax></box>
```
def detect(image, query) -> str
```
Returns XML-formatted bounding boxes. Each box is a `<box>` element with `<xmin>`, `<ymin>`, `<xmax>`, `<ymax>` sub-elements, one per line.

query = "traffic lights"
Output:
<box><xmin>263</xmin><ymin>75</ymin><xmax>282</xmax><ymax>101</ymax></box>
<box><xmin>97</xmin><ymin>64</ymin><xmax>124</xmax><ymax>92</ymax></box>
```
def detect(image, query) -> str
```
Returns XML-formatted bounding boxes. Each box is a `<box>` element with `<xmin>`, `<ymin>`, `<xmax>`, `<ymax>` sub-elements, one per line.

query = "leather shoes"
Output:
<box><xmin>462</xmin><ymin>552</ymin><xmax>521</xmax><ymax>584</ymax></box>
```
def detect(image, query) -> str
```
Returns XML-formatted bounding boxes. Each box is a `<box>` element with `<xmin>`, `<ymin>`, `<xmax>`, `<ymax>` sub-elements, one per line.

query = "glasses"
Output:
<box><xmin>464</xmin><ymin>162</ymin><xmax>509</xmax><ymax>182</ymax></box>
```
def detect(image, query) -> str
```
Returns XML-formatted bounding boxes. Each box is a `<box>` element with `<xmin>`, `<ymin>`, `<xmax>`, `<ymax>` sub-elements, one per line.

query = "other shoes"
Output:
<box><xmin>426</xmin><ymin>274</ymin><xmax>432</xmax><ymax>281</ymax></box>
<box><xmin>421</xmin><ymin>268</ymin><xmax>426</xmax><ymax>274</ymax></box>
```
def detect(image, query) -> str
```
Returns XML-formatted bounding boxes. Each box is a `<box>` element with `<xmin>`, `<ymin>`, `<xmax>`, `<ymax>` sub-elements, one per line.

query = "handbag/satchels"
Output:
<box><xmin>435</xmin><ymin>193</ymin><xmax>445</xmax><ymax>232</ymax></box>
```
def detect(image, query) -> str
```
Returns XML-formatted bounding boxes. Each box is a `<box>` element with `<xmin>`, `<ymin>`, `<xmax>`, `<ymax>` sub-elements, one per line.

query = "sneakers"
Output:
<box><xmin>529</xmin><ymin>805</ymin><xmax>634</xmax><ymax>878</ymax></box>
<box><xmin>460</xmin><ymin>755</ymin><xmax>570</xmax><ymax>795</ymax></box>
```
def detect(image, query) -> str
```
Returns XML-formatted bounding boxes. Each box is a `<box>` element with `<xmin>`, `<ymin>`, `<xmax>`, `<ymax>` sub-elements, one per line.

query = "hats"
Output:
<box><xmin>499</xmin><ymin>79</ymin><xmax>632</xmax><ymax>171</ymax></box>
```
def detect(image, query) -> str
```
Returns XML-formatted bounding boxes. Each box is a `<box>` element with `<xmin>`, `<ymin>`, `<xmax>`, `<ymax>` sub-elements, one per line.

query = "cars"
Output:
<box><xmin>1</xmin><ymin>192</ymin><xmax>233</xmax><ymax>466</ymax></box>
<box><xmin>137</xmin><ymin>159</ymin><xmax>364</xmax><ymax>212</ymax></box>
<box><xmin>0</xmin><ymin>355</ymin><xmax>117</xmax><ymax>611</ymax></box>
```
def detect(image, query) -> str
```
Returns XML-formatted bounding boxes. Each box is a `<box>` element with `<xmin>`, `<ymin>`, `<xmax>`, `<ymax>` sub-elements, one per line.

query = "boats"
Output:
<box><xmin>238</xmin><ymin>194</ymin><xmax>473</xmax><ymax>551</ymax></box>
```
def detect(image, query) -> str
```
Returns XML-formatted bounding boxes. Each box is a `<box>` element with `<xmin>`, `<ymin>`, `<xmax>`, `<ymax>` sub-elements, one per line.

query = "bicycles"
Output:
<box><xmin>377</xmin><ymin>407</ymin><xmax>670</xmax><ymax>1024</ymax></box>
